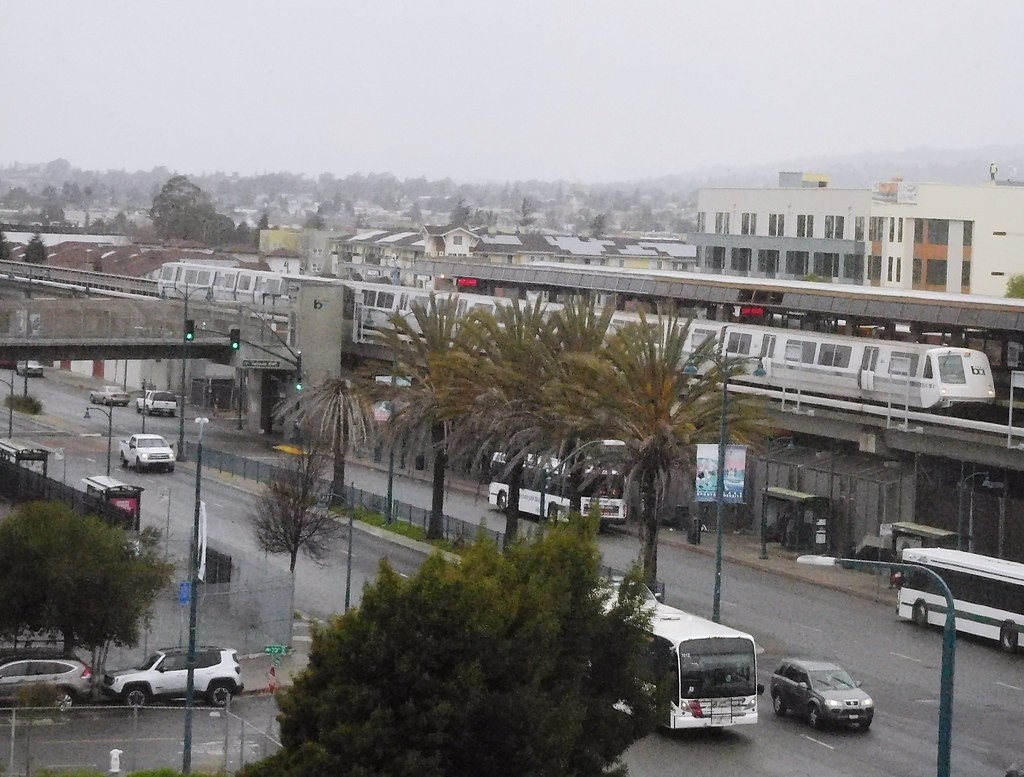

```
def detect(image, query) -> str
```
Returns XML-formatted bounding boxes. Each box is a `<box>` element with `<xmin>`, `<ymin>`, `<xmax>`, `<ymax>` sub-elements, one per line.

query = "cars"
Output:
<box><xmin>15</xmin><ymin>360</ymin><xmax>44</xmax><ymax>377</ymax></box>
<box><xmin>89</xmin><ymin>386</ymin><xmax>130</xmax><ymax>406</ymax></box>
<box><xmin>136</xmin><ymin>390</ymin><xmax>177</xmax><ymax>416</ymax></box>
<box><xmin>0</xmin><ymin>651</ymin><xmax>94</xmax><ymax>710</ymax></box>
<box><xmin>770</xmin><ymin>658</ymin><xmax>874</xmax><ymax>728</ymax></box>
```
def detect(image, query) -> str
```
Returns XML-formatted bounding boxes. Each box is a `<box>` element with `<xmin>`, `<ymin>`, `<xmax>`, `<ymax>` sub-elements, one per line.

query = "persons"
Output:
<box><xmin>990</xmin><ymin>162</ymin><xmax>998</xmax><ymax>180</ymax></box>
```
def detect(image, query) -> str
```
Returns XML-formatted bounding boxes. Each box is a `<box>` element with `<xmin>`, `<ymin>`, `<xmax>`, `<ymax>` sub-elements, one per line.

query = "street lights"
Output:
<box><xmin>797</xmin><ymin>555</ymin><xmax>956</xmax><ymax>777</ymax></box>
<box><xmin>161</xmin><ymin>282</ymin><xmax>214</xmax><ymax>462</ymax></box>
<box><xmin>8</xmin><ymin>268</ymin><xmax>50</xmax><ymax>396</ymax></box>
<box><xmin>84</xmin><ymin>398</ymin><xmax>113</xmax><ymax>475</ymax></box>
<box><xmin>540</xmin><ymin>439</ymin><xmax>626</xmax><ymax>526</ymax></box>
<box><xmin>682</xmin><ymin>348</ymin><xmax>767</xmax><ymax>623</ymax></box>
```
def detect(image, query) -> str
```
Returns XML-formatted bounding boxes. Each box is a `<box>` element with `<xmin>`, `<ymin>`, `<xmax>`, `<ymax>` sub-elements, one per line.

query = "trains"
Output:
<box><xmin>158</xmin><ymin>261</ymin><xmax>996</xmax><ymax>415</ymax></box>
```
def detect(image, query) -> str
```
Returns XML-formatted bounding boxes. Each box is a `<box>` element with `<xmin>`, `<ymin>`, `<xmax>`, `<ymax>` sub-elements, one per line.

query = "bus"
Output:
<box><xmin>489</xmin><ymin>452</ymin><xmax>628</xmax><ymax>530</ymax></box>
<box><xmin>894</xmin><ymin>547</ymin><xmax>1024</xmax><ymax>654</ymax></box>
<box><xmin>589</xmin><ymin>576</ymin><xmax>765</xmax><ymax>737</ymax></box>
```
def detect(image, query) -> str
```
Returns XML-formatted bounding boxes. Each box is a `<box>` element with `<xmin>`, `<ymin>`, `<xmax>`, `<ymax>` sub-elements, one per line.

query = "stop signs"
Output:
<box><xmin>268</xmin><ymin>667</ymin><xmax>276</xmax><ymax>694</ymax></box>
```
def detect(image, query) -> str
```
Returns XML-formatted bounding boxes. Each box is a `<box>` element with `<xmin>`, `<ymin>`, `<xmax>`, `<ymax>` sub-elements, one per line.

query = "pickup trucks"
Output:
<box><xmin>118</xmin><ymin>433</ymin><xmax>175</xmax><ymax>473</ymax></box>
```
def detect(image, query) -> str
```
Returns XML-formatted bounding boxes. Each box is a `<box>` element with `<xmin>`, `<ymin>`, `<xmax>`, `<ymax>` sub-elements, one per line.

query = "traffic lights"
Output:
<box><xmin>296</xmin><ymin>383</ymin><xmax>302</xmax><ymax>391</ymax></box>
<box><xmin>231</xmin><ymin>329</ymin><xmax>240</xmax><ymax>350</ymax></box>
<box><xmin>185</xmin><ymin>320</ymin><xmax>194</xmax><ymax>341</ymax></box>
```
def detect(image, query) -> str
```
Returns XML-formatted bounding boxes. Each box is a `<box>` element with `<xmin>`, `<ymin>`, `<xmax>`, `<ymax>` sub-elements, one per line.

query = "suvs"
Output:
<box><xmin>102</xmin><ymin>645</ymin><xmax>244</xmax><ymax>708</ymax></box>
